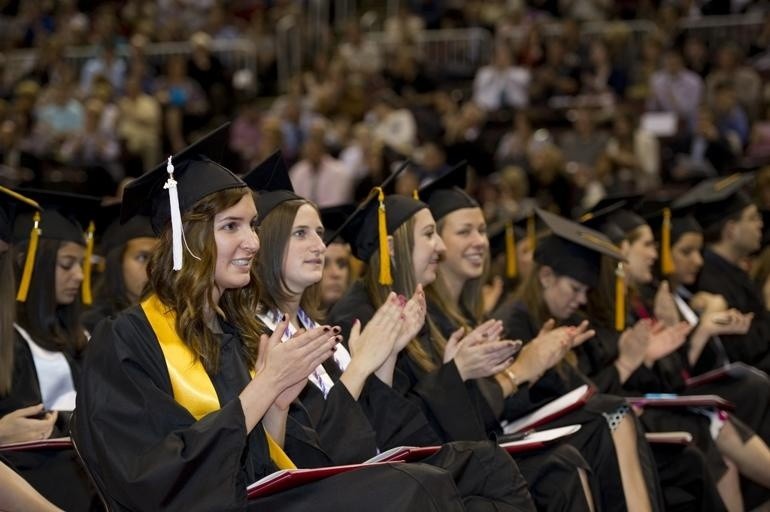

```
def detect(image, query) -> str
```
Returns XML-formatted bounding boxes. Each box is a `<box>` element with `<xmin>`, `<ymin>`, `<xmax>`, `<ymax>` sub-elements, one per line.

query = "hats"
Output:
<box><xmin>489</xmin><ymin>210</ymin><xmax>529</xmax><ymax>277</ymax></box>
<box><xmin>537</xmin><ymin>173</ymin><xmax>758</xmax><ymax>330</ymax></box>
<box><xmin>1</xmin><ymin>120</ymin><xmax>305</xmax><ymax>306</ymax></box>
<box><xmin>327</xmin><ymin>157</ymin><xmax>478</xmax><ymax>284</ymax></box>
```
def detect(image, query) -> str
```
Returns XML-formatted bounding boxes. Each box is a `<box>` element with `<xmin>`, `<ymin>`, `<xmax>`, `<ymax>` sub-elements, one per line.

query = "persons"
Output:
<box><xmin>2</xmin><ymin>1</ymin><xmax>770</xmax><ymax>512</ymax></box>
